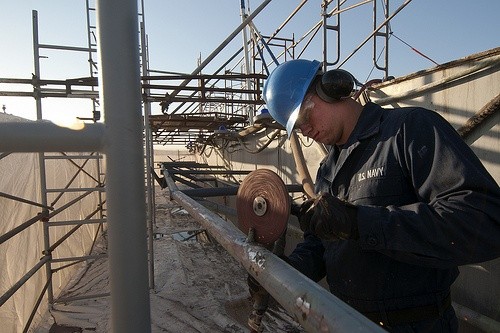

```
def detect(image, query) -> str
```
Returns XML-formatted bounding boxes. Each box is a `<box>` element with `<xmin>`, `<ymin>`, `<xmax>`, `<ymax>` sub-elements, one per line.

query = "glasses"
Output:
<box><xmin>295</xmin><ymin>90</ymin><xmax>316</xmax><ymax>132</ymax></box>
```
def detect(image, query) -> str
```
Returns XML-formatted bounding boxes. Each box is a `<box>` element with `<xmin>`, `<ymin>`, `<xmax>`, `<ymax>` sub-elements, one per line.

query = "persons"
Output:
<box><xmin>247</xmin><ymin>59</ymin><xmax>500</xmax><ymax>333</ymax></box>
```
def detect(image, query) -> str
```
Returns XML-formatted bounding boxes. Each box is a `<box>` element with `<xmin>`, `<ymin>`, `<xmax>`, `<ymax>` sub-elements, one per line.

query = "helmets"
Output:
<box><xmin>263</xmin><ymin>59</ymin><xmax>323</xmax><ymax>140</ymax></box>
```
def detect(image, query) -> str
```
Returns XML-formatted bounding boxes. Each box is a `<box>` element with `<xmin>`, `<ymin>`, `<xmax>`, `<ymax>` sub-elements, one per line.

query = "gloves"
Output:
<box><xmin>297</xmin><ymin>193</ymin><xmax>357</xmax><ymax>245</ymax></box>
